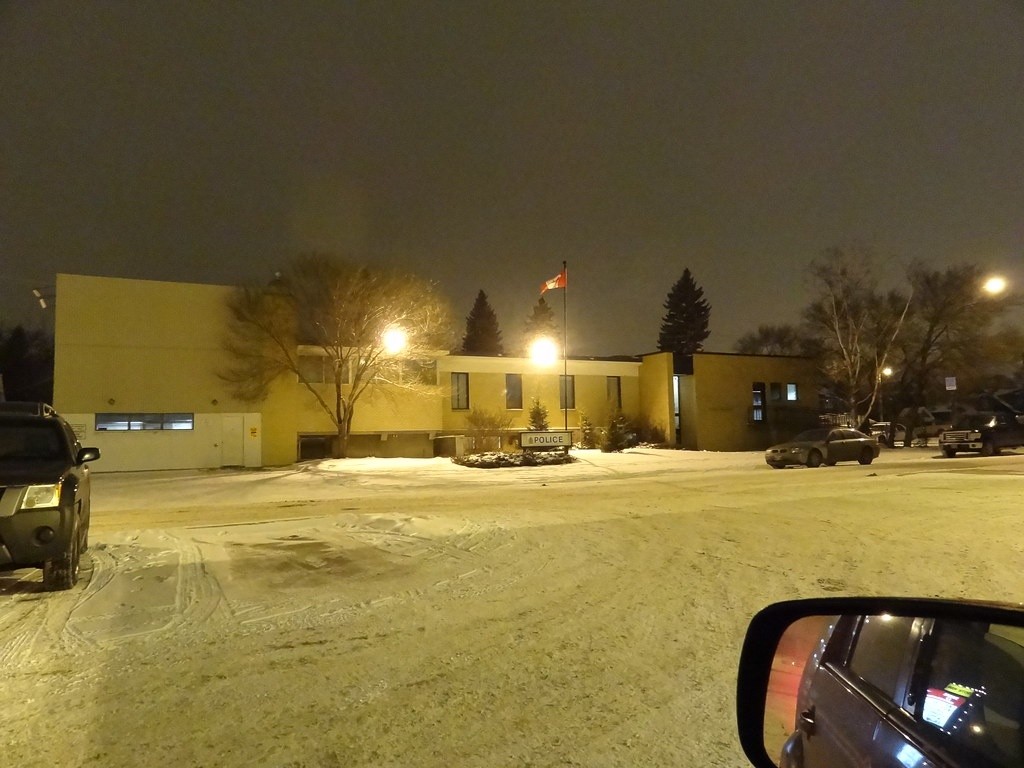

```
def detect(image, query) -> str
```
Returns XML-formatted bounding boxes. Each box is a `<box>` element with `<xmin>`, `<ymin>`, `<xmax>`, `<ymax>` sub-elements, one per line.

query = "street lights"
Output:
<box><xmin>879</xmin><ymin>368</ymin><xmax>892</xmax><ymax>422</ymax></box>
<box><xmin>949</xmin><ymin>277</ymin><xmax>1006</xmax><ymax>410</ymax></box>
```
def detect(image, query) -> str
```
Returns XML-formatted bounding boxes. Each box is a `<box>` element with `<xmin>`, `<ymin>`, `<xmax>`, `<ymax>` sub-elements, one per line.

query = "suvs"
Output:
<box><xmin>939</xmin><ymin>411</ymin><xmax>1024</xmax><ymax>458</ymax></box>
<box><xmin>0</xmin><ymin>402</ymin><xmax>101</xmax><ymax>591</ymax></box>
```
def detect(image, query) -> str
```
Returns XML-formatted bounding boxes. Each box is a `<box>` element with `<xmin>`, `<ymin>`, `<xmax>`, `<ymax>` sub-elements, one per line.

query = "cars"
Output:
<box><xmin>869</xmin><ymin>422</ymin><xmax>916</xmax><ymax>443</ymax></box>
<box><xmin>776</xmin><ymin>617</ymin><xmax>1023</xmax><ymax>768</ymax></box>
<box><xmin>913</xmin><ymin>421</ymin><xmax>954</xmax><ymax>436</ymax></box>
<box><xmin>766</xmin><ymin>426</ymin><xmax>881</xmax><ymax>467</ymax></box>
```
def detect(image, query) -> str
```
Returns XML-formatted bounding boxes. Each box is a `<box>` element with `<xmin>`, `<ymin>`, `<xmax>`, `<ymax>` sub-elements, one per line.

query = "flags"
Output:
<box><xmin>540</xmin><ymin>268</ymin><xmax>567</xmax><ymax>296</ymax></box>
